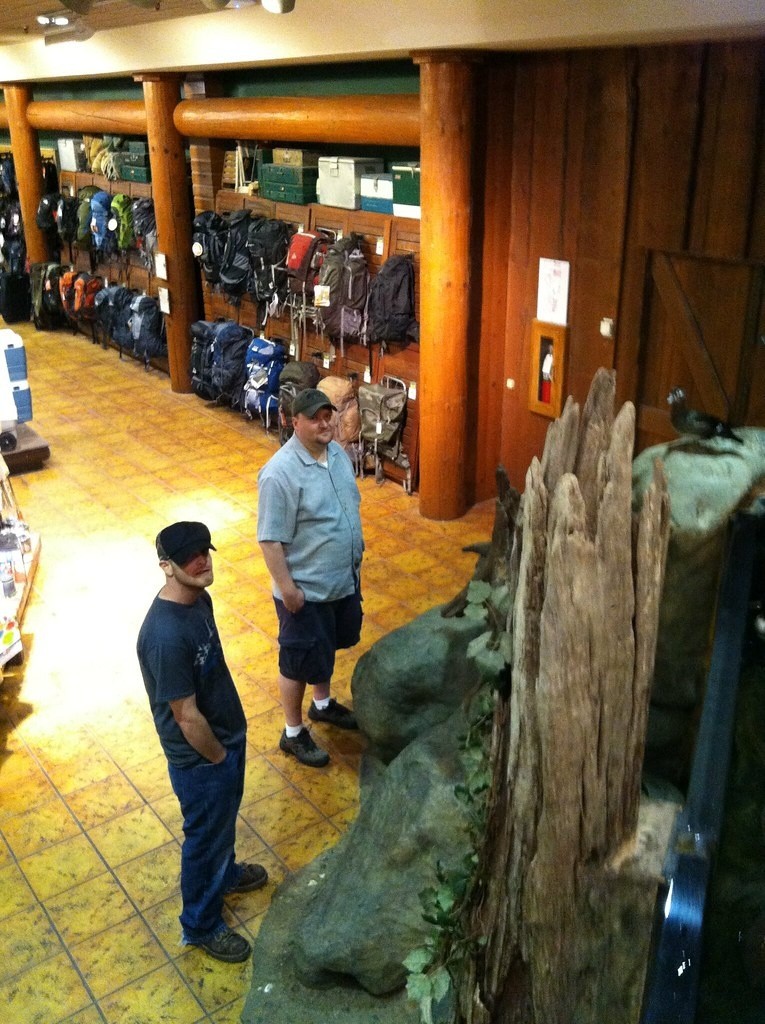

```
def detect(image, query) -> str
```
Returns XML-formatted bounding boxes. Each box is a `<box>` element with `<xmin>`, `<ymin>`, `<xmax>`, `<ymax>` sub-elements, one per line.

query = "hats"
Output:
<box><xmin>292</xmin><ymin>387</ymin><xmax>338</xmax><ymax>417</ymax></box>
<box><xmin>157</xmin><ymin>520</ymin><xmax>216</xmax><ymax>563</ymax></box>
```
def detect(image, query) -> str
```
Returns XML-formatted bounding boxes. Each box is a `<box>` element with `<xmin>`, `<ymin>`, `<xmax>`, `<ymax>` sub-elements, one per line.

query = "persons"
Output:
<box><xmin>257</xmin><ymin>388</ymin><xmax>366</xmax><ymax>768</ymax></box>
<box><xmin>137</xmin><ymin>521</ymin><xmax>268</xmax><ymax>962</ymax></box>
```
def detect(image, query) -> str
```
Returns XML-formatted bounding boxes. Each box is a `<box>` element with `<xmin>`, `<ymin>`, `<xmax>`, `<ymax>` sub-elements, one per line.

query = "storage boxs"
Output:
<box><xmin>119</xmin><ymin>141</ymin><xmax>153</xmax><ymax>183</ymax></box>
<box><xmin>247</xmin><ymin>147</ymin><xmax>422</xmax><ymax>220</ymax></box>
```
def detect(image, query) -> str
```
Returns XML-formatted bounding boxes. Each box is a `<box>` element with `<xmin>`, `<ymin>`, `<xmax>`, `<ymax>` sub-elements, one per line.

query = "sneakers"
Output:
<box><xmin>279</xmin><ymin>728</ymin><xmax>330</xmax><ymax>766</ymax></box>
<box><xmin>234</xmin><ymin>861</ymin><xmax>269</xmax><ymax>890</ymax></box>
<box><xmin>202</xmin><ymin>927</ymin><xmax>252</xmax><ymax>962</ymax></box>
<box><xmin>308</xmin><ymin>698</ymin><xmax>358</xmax><ymax>728</ymax></box>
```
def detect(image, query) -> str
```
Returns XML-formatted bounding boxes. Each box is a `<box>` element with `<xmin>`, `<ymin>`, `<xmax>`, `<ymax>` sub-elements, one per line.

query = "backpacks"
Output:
<box><xmin>0</xmin><ymin>155</ymin><xmax>420</xmax><ymax>478</ymax></box>
